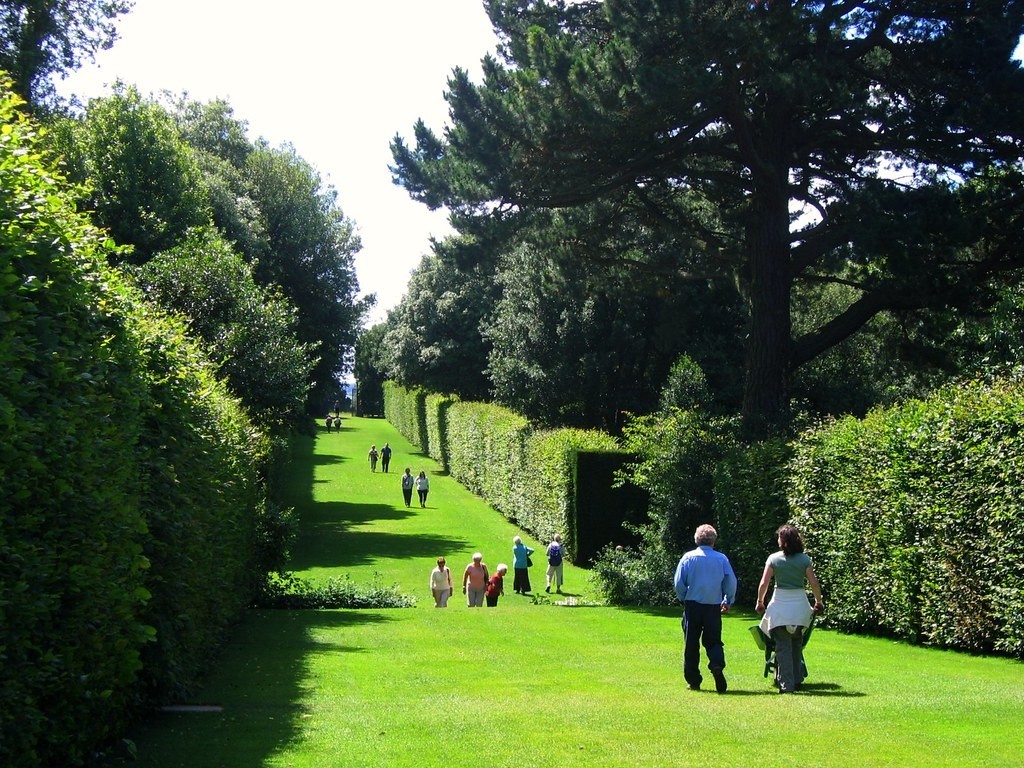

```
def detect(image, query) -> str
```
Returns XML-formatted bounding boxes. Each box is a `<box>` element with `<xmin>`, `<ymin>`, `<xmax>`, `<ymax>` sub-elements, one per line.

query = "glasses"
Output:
<box><xmin>439</xmin><ymin>565</ymin><xmax>444</xmax><ymax>568</ymax></box>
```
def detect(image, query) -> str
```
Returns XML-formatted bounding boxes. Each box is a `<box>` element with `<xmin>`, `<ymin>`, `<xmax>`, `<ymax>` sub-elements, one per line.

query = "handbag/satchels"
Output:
<box><xmin>526</xmin><ymin>547</ymin><xmax>533</xmax><ymax>567</ymax></box>
<box><xmin>482</xmin><ymin>565</ymin><xmax>490</xmax><ymax>591</ymax></box>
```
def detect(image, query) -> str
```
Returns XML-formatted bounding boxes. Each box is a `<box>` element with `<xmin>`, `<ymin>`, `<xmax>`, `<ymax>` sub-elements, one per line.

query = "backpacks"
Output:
<box><xmin>548</xmin><ymin>543</ymin><xmax>563</xmax><ymax>566</ymax></box>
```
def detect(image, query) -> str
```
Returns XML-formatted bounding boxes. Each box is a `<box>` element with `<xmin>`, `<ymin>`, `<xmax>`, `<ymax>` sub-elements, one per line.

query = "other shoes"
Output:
<box><xmin>522</xmin><ymin>590</ymin><xmax>525</xmax><ymax>595</ymax></box>
<box><xmin>557</xmin><ymin>590</ymin><xmax>562</xmax><ymax>594</ymax></box>
<box><xmin>516</xmin><ymin>590</ymin><xmax>520</xmax><ymax>594</ymax></box>
<box><xmin>712</xmin><ymin>667</ymin><xmax>727</xmax><ymax>693</ymax></box>
<box><xmin>687</xmin><ymin>684</ymin><xmax>701</xmax><ymax>690</ymax></box>
<box><xmin>545</xmin><ymin>586</ymin><xmax>550</xmax><ymax>593</ymax></box>
<box><xmin>778</xmin><ymin>686</ymin><xmax>793</xmax><ymax>693</ymax></box>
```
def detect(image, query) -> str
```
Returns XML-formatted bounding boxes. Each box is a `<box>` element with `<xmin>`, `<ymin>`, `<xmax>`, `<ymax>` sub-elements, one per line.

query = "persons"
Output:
<box><xmin>368</xmin><ymin>444</ymin><xmax>380</xmax><ymax>473</ymax></box>
<box><xmin>401</xmin><ymin>467</ymin><xmax>414</xmax><ymax>508</ymax></box>
<box><xmin>545</xmin><ymin>533</ymin><xmax>566</xmax><ymax>594</ymax></box>
<box><xmin>485</xmin><ymin>563</ymin><xmax>508</xmax><ymax>606</ymax></box>
<box><xmin>414</xmin><ymin>471</ymin><xmax>429</xmax><ymax>508</ymax></box>
<box><xmin>512</xmin><ymin>536</ymin><xmax>534</xmax><ymax>595</ymax></box>
<box><xmin>463</xmin><ymin>552</ymin><xmax>490</xmax><ymax>607</ymax></box>
<box><xmin>380</xmin><ymin>442</ymin><xmax>392</xmax><ymax>473</ymax></box>
<box><xmin>431</xmin><ymin>556</ymin><xmax>453</xmax><ymax>608</ymax></box>
<box><xmin>334</xmin><ymin>400</ymin><xmax>341</xmax><ymax>416</ymax></box>
<box><xmin>674</xmin><ymin>523</ymin><xmax>737</xmax><ymax>694</ymax></box>
<box><xmin>334</xmin><ymin>416</ymin><xmax>341</xmax><ymax>433</ymax></box>
<box><xmin>326</xmin><ymin>415</ymin><xmax>332</xmax><ymax>435</ymax></box>
<box><xmin>755</xmin><ymin>525</ymin><xmax>825</xmax><ymax>694</ymax></box>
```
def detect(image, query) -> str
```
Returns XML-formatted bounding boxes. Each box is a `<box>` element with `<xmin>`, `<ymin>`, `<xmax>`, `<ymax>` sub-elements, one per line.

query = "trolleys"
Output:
<box><xmin>748</xmin><ymin>607</ymin><xmax>817</xmax><ymax>685</ymax></box>
